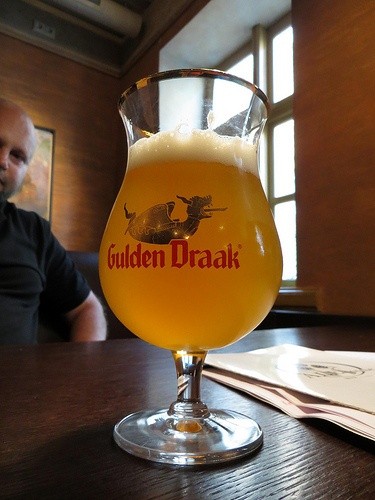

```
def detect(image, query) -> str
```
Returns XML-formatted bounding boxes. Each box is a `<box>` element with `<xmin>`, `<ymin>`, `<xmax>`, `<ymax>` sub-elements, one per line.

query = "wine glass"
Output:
<box><xmin>99</xmin><ymin>69</ymin><xmax>283</xmax><ymax>467</ymax></box>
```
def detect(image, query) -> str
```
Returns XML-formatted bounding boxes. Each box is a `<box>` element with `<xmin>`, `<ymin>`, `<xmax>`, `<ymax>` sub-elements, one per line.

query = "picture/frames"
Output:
<box><xmin>5</xmin><ymin>124</ymin><xmax>55</xmax><ymax>228</ymax></box>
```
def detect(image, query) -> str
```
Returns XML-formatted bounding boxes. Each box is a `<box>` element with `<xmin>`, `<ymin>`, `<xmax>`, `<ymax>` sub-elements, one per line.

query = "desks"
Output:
<box><xmin>0</xmin><ymin>323</ymin><xmax>374</xmax><ymax>500</ymax></box>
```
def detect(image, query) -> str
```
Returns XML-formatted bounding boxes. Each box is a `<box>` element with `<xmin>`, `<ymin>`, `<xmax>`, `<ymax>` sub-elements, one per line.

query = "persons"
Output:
<box><xmin>0</xmin><ymin>96</ymin><xmax>108</xmax><ymax>347</ymax></box>
